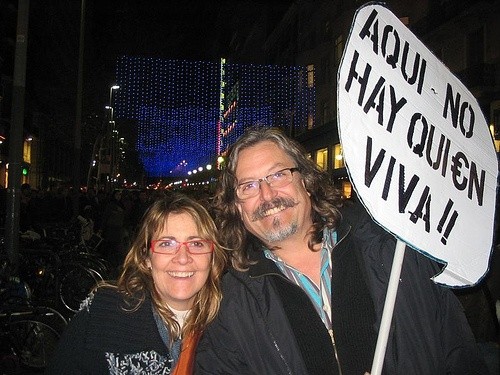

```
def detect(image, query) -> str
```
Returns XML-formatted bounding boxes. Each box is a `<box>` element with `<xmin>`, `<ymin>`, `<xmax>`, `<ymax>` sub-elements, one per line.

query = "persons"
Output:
<box><xmin>1</xmin><ymin>170</ymin><xmax>217</xmax><ymax>282</ymax></box>
<box><xmin>44</xmin><ymin>191</ymin><xmax>235</xmax><ymax>374</ymax></box>
<box><xmin>191</xmin><ymin>125</ymin><xmax>490</xmax><ymax>374</ymax></box>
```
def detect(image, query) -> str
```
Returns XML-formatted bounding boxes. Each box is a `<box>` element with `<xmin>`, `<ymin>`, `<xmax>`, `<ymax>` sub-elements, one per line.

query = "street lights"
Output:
<box><xmin>104</xmin><ymin>85</ymin><xmax>126</xmax><ymax>189</ymax></box>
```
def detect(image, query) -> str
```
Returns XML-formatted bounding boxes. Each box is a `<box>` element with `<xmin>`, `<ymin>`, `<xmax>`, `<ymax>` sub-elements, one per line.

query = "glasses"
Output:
<box><xmin>229</xmin><ymin>165</ymin><xmax>301</xmax><ymax>204</ymax></box>
<box><xmin>150</xmin><ymin>236</ymin><xmax>214</xmax><ymax>256</ymax></box>
